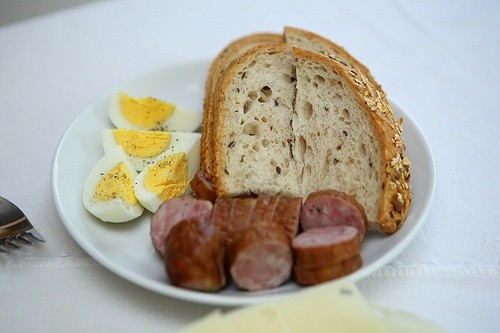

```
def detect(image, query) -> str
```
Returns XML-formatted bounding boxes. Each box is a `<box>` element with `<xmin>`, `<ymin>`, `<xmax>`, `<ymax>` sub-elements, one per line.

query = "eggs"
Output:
<box><xmin>83</xmin><ymin>88</ymin><xmax>203</xmax><ymax>224</ymax></box>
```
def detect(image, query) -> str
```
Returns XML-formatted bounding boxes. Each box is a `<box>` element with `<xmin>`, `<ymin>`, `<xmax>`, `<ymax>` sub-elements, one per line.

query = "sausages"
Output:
<box><xmin>149</xmin><ymin>169</ymin><xmax>369</xmax><ymax>293</ymax></box>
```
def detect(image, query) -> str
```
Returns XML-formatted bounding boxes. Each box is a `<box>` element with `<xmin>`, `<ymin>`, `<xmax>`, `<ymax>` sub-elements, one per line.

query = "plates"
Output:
<box><xmin>50</xmin><ymin>58</ymin><xmax>438</xmax><ymax>304</ymax></box>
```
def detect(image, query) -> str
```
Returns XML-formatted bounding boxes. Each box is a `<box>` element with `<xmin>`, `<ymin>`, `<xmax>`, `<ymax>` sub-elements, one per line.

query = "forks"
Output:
<box><xmin>1</xmin><ymin>191</ymin><xmax>46</xmax><ymax>267</ymax></box>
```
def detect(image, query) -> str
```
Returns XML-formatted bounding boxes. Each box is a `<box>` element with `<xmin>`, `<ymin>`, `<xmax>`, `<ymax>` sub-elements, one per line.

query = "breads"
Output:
<box><xmin>198</xmin><ymin>26</ymin><xmax>412</xmax><ymax>236</ymax></box>
